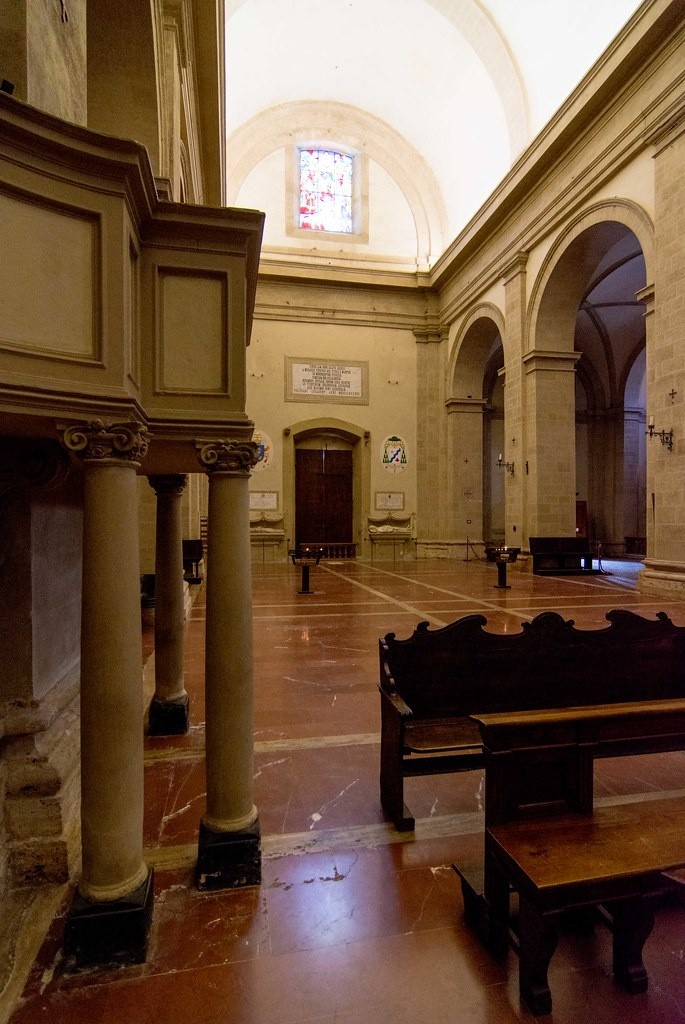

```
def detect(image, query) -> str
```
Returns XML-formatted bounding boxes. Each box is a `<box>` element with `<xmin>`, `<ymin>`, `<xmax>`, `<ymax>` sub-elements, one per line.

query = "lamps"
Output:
<box><xmin>496</xmin><ymin>454</ymin><xmax>514</xmax><ymax>477</ymax></box>
<box><xmin>645</xmin><ymin>416</ymin><xmax>673</xmax><ymax>451</ymax></box>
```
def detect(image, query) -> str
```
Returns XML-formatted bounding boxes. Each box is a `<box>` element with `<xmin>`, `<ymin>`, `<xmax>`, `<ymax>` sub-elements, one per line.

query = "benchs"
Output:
<box><xmin>453</xmin><ymin>699</ymin><xmax>685</xmax><ymax>1014</ymax></box>
<box><xmin>376</xmin><ymin>609</ymin><xmax>685</xmax><ymax>832</ymax></box>
